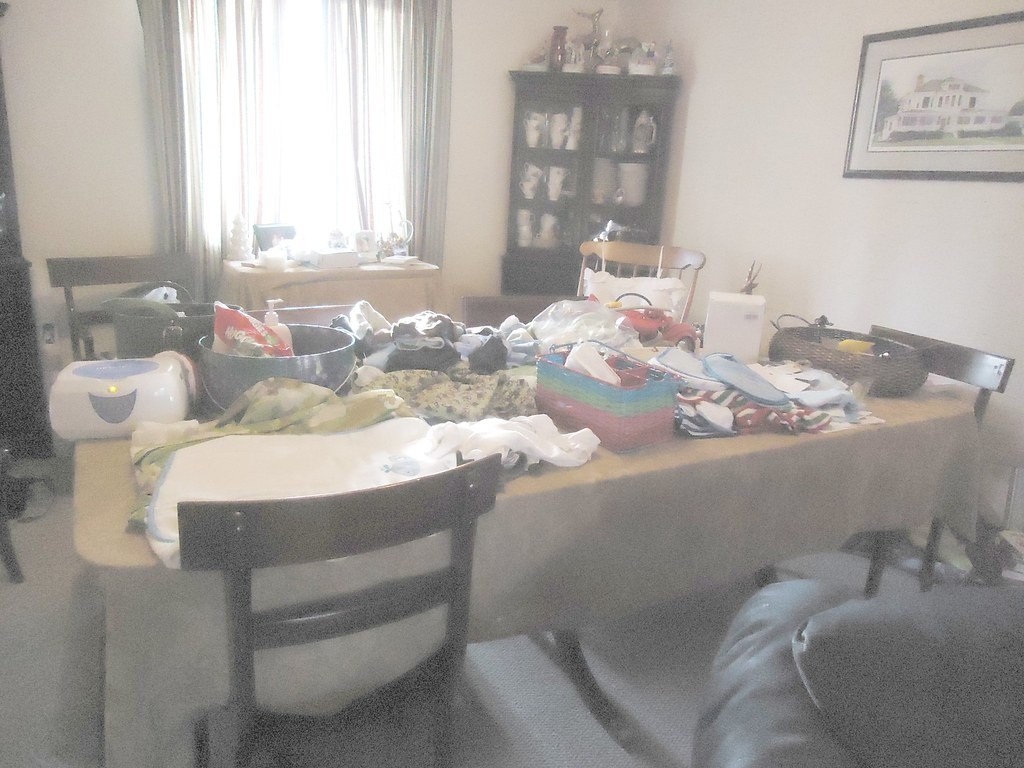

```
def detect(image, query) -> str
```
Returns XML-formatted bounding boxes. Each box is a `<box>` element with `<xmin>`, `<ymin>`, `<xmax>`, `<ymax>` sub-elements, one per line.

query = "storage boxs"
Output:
<box><xmin>701</xmin><ymin>291</ymin><xmax>765</xmax><ymax>365</ymax></box>
<box><xmin>382</xmin><ymin>255</ymin><xmax>419</xmax><ymax>265</ymax></box>
<box><xmin>309</xmin><ymin>249</ymin><xmax>359</xmax><ymax>269</ymax></box>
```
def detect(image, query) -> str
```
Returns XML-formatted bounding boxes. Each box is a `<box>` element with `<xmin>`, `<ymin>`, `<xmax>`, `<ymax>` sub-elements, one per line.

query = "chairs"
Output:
<box><xmin>178</xmin><ymin>450</ymin><xmax>503</xmax><ymax>768</ymax></box>
<box><xmin>254</xmin><ymin>223</ymin><xmax>296</xmax><ymax>260</ymax></box>
<box><xmin>754</xmin><ymin>322</ymin><xmax>1016</xmax><ymax>598</ymax></box>
<box><xmin>47</xmin><ymin>256</ymin><xmax>185</xmax><ymax>362</ymax></box>
<box><xmin>244</xmin><ymin>305</ymin><xmax>353</xmax><ymax>327</ymax></box>
<box><xmin>575</xmin><ymin>239</ymin><xmax>708</xmax><ymax>328</ymax></box>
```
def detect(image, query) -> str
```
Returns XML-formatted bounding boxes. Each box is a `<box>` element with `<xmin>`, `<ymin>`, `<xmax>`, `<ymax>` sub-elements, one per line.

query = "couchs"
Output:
<box><xmin>691</xmin><ymin>574</ymin><xmax>1024</xmax><ymax>768</ymax></box>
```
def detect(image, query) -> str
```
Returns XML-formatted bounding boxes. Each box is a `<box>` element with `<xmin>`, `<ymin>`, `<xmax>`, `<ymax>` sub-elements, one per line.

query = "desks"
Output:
<box><xmin>73</xmin><ymin>354</ymin><xmax>981</xmax><ymax>768</ymax></box>
<box><xmin>221</xmin><ymin>256</ymin><xmax>440</xmax><ymax>322</ymax></box>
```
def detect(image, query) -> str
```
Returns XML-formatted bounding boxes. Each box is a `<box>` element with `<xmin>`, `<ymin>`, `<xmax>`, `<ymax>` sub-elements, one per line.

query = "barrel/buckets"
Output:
<box><xmin>199</xmin><ymin>325</ymin><xmax>359</xmax><ymax>416</ymax></box>
<box><xmin>108</xmin><ymin>304</ymin><xmax>242</xmax><ymax>363</ymax></box>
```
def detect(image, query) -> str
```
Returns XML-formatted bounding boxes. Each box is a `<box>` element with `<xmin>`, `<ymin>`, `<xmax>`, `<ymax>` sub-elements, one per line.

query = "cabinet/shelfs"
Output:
<box><xmin>504</xmin><ymin>72</ymin><xmax>686</xmax><ymax>303</ymax></box>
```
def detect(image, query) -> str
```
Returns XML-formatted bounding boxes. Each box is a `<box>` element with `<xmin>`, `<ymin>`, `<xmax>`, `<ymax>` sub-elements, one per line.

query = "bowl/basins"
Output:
<box><xmin>594</xmin><ymin>157</ymin><xmax>648</xmax><ymax>209</ymax></box>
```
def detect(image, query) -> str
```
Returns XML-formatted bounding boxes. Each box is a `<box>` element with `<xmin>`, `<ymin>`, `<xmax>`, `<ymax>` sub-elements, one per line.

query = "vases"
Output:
<box><xmin>549</xmin><ymin>26</ymin><xmax>568</xmax><ymax>71</ymax></box>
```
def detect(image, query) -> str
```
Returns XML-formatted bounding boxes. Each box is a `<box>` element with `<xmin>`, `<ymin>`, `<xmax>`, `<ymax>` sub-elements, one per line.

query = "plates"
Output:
<box><xmin>533</xmin><ymin>237</ymin><xmax>558</xmax><ymax>247</ymax></box>
<box><xmin>561</xmin><ymin>184</ymin><xmax>576</xmax><ymax>198</ymax></box>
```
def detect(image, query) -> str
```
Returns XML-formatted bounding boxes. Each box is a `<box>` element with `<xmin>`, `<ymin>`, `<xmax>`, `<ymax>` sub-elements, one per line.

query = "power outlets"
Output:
<box><xmin>43</xmin><ymin>323</ymin><xmax>56</xmax><ymax>344</ymax></box>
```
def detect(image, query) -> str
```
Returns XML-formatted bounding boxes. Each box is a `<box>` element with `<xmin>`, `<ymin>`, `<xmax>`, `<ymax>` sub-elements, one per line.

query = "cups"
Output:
<box><xmin>515</xmin><ymin>208</ymin><xmax>533</xmax><ymax>249</ymax></box>
<box><xmin>523</xmin><ymin>107</ymin><xmax>583</xmax><ymax>150</ymax></box>
<box><xmin>536</xmin><ymin>213</ymin><xmax>557</xmax><ymax>238</ymax></box>
<box><xmin>519</xmin><ymin>163</ymin><xmax>569</xmax><ymax>201</ymax></box>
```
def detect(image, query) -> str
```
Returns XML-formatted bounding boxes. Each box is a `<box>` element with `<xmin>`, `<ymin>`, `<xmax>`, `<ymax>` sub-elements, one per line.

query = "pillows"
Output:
<box><xmin>791</xmin><ymin>583</ymin><xmax>1024</xmax><ymax>768</ymax></box>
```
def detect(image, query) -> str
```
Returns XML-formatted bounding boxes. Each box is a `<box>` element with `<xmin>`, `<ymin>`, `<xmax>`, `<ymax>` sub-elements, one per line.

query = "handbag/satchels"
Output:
<box><xmin>584</xmin><ymin>266</ymin><xmax>684</xmax><ymax>316</ymax></box>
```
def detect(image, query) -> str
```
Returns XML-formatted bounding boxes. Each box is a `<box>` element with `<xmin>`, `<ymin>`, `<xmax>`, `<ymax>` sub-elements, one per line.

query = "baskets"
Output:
<box><xmin>768</xmin><ymin>314</ymin><xmax>944</xmax><ymax>398</ymax></box>
<box><xmin>535</xmin><ymin>340</ymin><xmax>687</xmax><ymax>454</ymax></box>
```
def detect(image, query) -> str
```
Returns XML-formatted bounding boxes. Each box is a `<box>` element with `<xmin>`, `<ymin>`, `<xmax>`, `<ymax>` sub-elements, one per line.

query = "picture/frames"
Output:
<box><xmin>840</xmin><ymin>10</ymin><xmax>1024</xmax><ymax>181</ymax></box>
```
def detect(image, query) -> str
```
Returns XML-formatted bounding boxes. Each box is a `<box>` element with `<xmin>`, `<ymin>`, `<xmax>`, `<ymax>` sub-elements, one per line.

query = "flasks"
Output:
<box><xmin>632</xmin><ymin>109</ymin><xmax>659</xmax><ymax>154</ymax></box>
